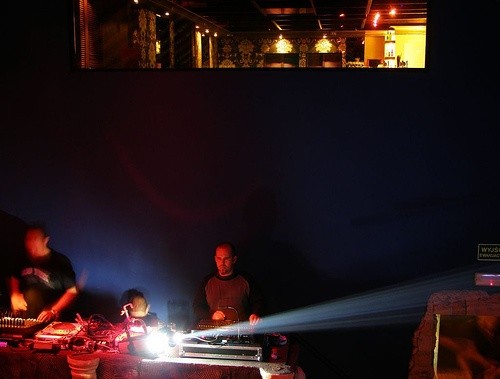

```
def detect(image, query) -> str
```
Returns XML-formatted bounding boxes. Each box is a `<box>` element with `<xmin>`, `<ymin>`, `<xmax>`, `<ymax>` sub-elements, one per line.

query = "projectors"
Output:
<box><xmin>473</xmin><ymin>273</ymin><xmax>500</xmax><ymax>287</ymax></box>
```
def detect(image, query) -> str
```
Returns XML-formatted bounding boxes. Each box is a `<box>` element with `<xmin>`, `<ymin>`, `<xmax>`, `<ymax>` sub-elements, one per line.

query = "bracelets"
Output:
<box><xmin>51</xmin><ymin>310</ymin><xmax>56</xmax><ymax>314</ymax></box>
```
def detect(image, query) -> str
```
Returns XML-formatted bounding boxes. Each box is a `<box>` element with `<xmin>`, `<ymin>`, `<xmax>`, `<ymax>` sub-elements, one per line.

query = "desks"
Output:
<box><xmin>0</xmin><ymin>343</ymin><xmax>306</xmax><ymax>379</ymax></box>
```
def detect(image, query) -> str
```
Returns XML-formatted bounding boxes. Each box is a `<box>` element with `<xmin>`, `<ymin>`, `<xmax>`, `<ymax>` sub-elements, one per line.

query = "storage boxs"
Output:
<box><xmin>177</xmin><ymin>343</ymin><xmax>263</xmax><ymax>362</ymax></box>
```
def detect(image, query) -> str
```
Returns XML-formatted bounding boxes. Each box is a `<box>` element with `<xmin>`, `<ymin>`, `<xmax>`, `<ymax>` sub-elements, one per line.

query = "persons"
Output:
<box><xmin>8</xmin><ymin>222</ymin><xmax>80</xmax><ymax>324</ymax></box>
<box><xmin>192</xmin><ymin>241</ymin><xmax>261</xmax><ymax>323</ymax></box>
<box><xmin>118</xmin><ymin>289</ymin><xmax>159</xmax><ymax>328</ymax></box>
<box><xmin>458</xmin><ymin>316</ymin><xmax>500</xmax><ymax>379</ymax></box>
